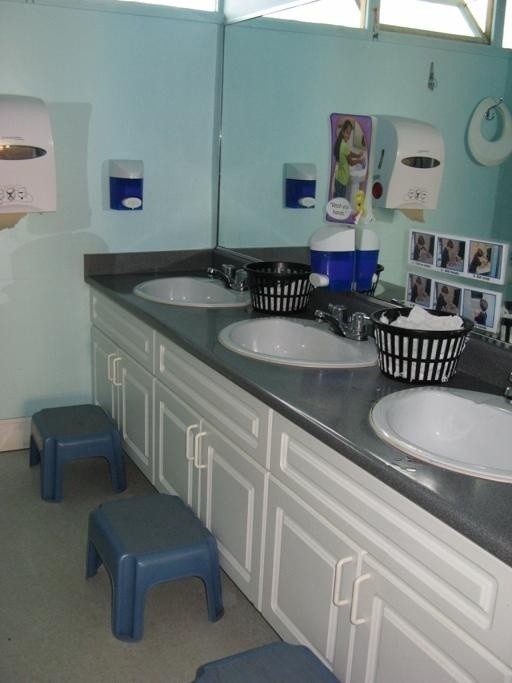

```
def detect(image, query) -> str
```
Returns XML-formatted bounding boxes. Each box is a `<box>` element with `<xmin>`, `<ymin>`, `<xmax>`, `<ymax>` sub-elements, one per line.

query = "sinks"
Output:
<box><xmin>218</xmin><ymin>317</ymin><xmax>378</xmax><ymax>368</ymax></box>
<box><xmin>132</xmin><ymin>276</ymin><xmax>249</xmax><ymax>309</ymax></box>
<box><xmin>374</xmin><ymin>283</ymin><xmax>385</xmax><ymax>296</ymax></box>
<box><xmin>369</xmin><ymin>386</ymin><xmax>511</xmax><ymax>485</ymax></box>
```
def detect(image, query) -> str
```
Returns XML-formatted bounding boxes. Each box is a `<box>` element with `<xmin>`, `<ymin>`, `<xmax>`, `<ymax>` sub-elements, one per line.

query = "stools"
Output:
<box><xmin>191</xmin><ymin>640</ymin><xmax>344</xmax><ymax>683</ymax></box>
<box><xmin>83</xmin><ymin>489</ymin><xmax>226</xmax><ymax>644</ymax></box>
<box><xmin>27</xmin><ymin>402</ymin><xmax>129</xmax><ymax>504</ymax></box>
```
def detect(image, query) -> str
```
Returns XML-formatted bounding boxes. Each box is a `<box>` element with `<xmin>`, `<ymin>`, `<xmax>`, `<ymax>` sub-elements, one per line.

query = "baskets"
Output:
<box><xmin>245</xmin><ymin>262</ymin><xmax>314</xmax><ymax>314</ymax></box>
<box><xmin>371</xmin><ymin>308</ymin><xmax>473</xmax><ymax>385</ymax></box>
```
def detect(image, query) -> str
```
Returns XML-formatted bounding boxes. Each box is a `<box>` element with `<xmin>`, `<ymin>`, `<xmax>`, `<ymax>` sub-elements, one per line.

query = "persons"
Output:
<box><xmin>410</xmin><ymin>233</ymin><xmax>490</xmax><ymax>327</ymax></box>
<box><xmin>333</xmin><ymin>120</ymin><xmax>365</xmax><ymax>200</ymax></box>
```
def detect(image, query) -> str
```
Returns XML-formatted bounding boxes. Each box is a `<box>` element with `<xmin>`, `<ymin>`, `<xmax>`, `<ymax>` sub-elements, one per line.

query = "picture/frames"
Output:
<box><xmin>403</xmin><ymin>227</ymin><xmax>510</xmax><ymax>336</ymax></box>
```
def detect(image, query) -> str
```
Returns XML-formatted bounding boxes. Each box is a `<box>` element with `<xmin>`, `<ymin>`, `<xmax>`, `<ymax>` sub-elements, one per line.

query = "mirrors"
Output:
<box><xmin>215</xmin><ymin>0</ymin><xmax>512</xmax><ymax>395</ymax></box>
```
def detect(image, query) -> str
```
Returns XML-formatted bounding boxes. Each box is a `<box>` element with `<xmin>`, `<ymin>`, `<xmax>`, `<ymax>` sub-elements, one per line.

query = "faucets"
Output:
<box><xmin>312</xmin><ymin>302</ymin><xmax>352</xmax><ymax>336</ymax></box>
<box><xmin>207</xmin><ymin>263</ymin><xmax>236</xmax><ymax>288</ymax></box>
<box><xmin>504</xmin><ymin>372</ymin><xmax>512</xmax><ymax>405</ymax></box>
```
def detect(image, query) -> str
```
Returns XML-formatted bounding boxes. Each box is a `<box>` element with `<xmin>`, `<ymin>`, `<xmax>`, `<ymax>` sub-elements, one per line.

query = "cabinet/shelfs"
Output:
<box><xmin>150</xmin><ymin>317</ymin><xmax>272</xmax><ymax>614</ymax></box>
<box><xmin>89</xmin><ymin>279</ymin><xmax>155</xmax><ymax>487</ymax></box>
<box><xmin>261</xmin><ymin>394</ymin><xmax>512</xmax><ymax>682</ymax></box>
